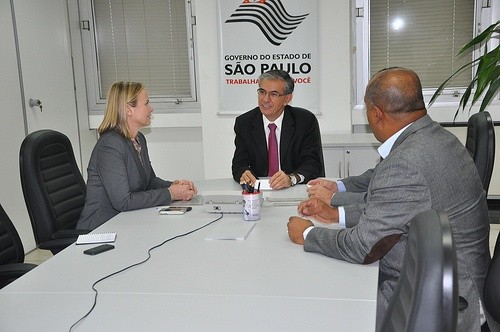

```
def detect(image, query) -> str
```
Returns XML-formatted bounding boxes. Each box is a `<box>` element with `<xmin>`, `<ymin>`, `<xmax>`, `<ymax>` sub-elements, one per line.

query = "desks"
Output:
<box><xmin>0</xmin><ymin>177</ymin><xmax>381</xmax><ymax>332</ymax></box>
<box><xmin>18</xmin><ymin>130</ymin><xmax>85</xmax><ymax>256</ymax></box>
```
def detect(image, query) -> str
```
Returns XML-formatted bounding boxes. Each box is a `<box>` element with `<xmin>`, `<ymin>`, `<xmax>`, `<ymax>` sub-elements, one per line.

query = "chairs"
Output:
<box><xmin>0</xmin><ymin>203</ymin><xmax>39</xmax><ymax>288</ymax></box>
<box><xmin>382</xmin><ymin>111</ymin><xmax>500</xmax><ymax>332</ymax></box>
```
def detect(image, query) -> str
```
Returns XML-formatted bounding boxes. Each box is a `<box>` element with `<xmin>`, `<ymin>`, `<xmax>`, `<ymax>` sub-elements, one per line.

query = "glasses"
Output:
<box><xmin>257</xmin><ymin>89</ymin><xmax>287</xmax><ymax>97</ymax></box>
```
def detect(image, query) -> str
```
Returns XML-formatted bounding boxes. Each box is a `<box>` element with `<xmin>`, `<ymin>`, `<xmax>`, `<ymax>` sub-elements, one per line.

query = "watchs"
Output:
<box><xmin>289</xmin><ymin>175</ymin><xmax>297</xmax><ymax>187</ymax></box>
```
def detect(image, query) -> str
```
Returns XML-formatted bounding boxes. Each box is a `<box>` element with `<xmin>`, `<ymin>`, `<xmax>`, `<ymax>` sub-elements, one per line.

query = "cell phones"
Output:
<box><xmin>159</xmin><ymin>207</ymin><xmax>193</xmax><ymax>214</ymax></box>
<box><xmin>84</xmin><ymin>244</ymin><xmax>115</xmax><ymax>256</ymax></box>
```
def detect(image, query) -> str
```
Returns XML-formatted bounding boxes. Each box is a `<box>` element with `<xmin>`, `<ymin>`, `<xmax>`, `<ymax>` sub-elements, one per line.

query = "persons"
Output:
<box><xmin>76</xmin><ymin>81</ymin><xmax>198</xmax><ymax>231</ymax></box>
<box><xmin>287</xmin><ymin>69</ymin><xmax>491</xmax><ymax>332</ymax></box>
<box><xmin>232</xmin><ymin>70</ymin><xmax>326</xmax><ymax>191</ymax></box>
<box><xmin>306</xmin><ymin>67</ymin><xmax>424</xmax><ymax>209</ymax></box>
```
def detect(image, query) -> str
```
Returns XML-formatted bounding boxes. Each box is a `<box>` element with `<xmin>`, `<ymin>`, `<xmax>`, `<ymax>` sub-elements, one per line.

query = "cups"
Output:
<box><xmin>242</xmin><ymin>189</ymin><xmax>262</xmax><ymax>220</ymax></box>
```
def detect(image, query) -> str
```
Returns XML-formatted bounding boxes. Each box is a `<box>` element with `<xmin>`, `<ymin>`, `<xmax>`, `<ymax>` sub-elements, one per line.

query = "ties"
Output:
<box><xmin>268</xmin><ymin>124</ymin><xmax>279</xmax><ymax>177</ymax></box>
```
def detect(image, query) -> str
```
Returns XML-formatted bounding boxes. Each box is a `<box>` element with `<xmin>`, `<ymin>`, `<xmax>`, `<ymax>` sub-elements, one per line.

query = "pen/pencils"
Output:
<box><xmin>239</xmin><ymin>182</ymin><xmax>255</xmax><ymax>193</ymax></box>
<box><xmin>257</xmin><ymin>182</ymin><xmax>261</xmax><ymax>193</ymax></box>
